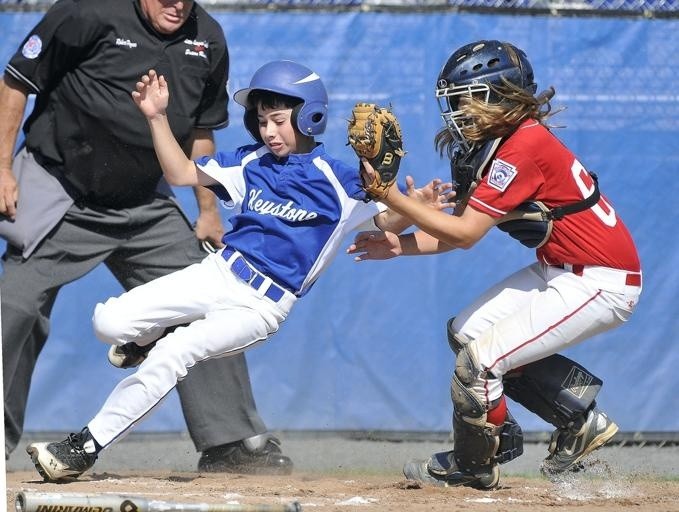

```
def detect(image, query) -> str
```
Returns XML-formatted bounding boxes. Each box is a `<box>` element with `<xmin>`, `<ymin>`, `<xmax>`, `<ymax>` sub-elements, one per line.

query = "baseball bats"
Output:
<box><xmin>15</xmin><ymin>489</ymin><xmax>301</xmax><ymax>512</ymax></box>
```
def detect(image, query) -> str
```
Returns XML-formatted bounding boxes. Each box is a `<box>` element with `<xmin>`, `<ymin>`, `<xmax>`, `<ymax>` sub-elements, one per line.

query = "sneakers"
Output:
<box><xmin>539</xmin><ymin>407</ymin><xmax>619</xmax><ymax>478</ymax></box>
<box><xmin>107</xmin><ymin>343</ymin><xmax>156</xmax><ymax>368</ymax></box>
<box><xmin>403</xmin><ymin>457</ymin><xmax>500</xmax><ymax>489</ymax></box>
<box><xmin>198</xmin><ymin>440</ymin><xmax>293</xmax><ymax>475</ymax></box>
<box><xmin>26</xmin><ymin>427</ymin><xmax>98</xmax><ymax>482</ymax></box>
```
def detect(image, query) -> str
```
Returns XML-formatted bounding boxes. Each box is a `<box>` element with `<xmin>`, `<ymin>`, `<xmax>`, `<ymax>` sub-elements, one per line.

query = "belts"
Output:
<box><xmin>574</xmin><ymin>264</ymin><xmax>641</xmax><ymax>286</ymax></box>
<box><xmin>223</xmin><ymin>245</ymin><xmax>284</xmax><ymax>302</ymax></box>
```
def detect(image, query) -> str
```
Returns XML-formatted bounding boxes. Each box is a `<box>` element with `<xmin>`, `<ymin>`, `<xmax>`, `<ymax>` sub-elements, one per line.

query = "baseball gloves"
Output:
<box><xmin>348</xmin><ymin>101</ymin><xmax>402</xmax><ymax>201</ymax></box>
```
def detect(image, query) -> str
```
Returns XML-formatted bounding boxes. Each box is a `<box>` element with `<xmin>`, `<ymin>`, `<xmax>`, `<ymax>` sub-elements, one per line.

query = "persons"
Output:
<box><xmin>346</xmin><ymin>39</ymin><xmax>643</xmax><ymax>490</ymax></box>
<box><xmin>0</xmin><ymin>0</ymin><xmax>294</xmax><ymax>477</ymax></box>
<box><xmin>27</xmin><ymin>60</ymin><xmax>457</xmax><ymax>484</ymax></box>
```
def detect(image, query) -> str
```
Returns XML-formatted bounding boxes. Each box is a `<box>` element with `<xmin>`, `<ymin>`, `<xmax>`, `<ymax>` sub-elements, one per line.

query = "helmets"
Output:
<box><xmin>435</xmin><ymin>40</ymin><xmax>537</xmax><ymax>155</ymax></box>
<box><xmin>234</xmin><ymin>60</ymin><xmax>328</xmax><ymax>143</ymax></box>
